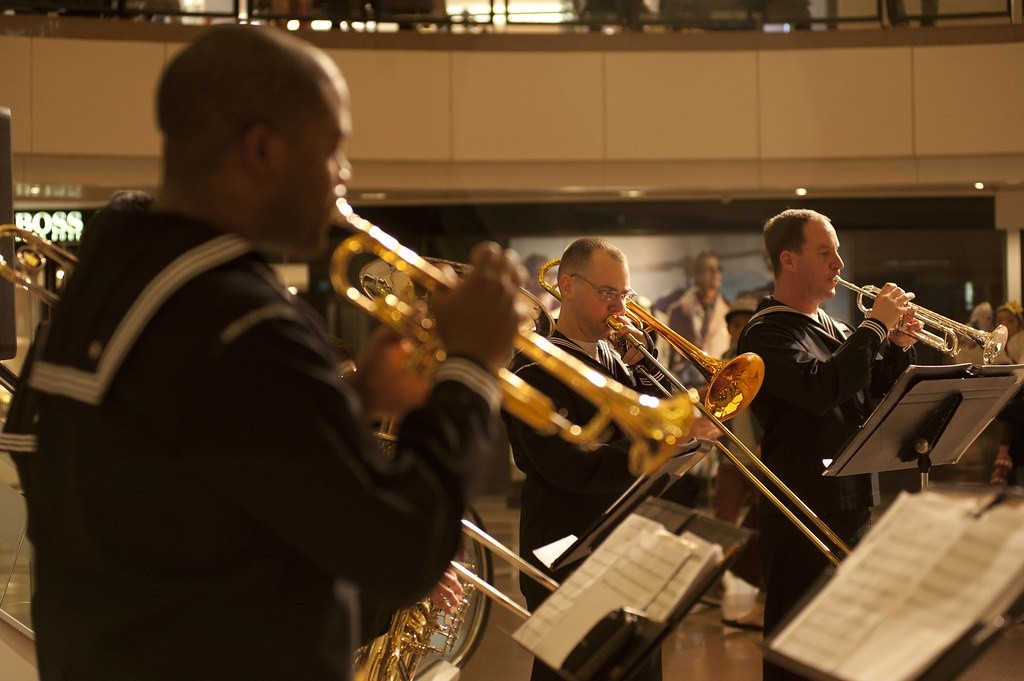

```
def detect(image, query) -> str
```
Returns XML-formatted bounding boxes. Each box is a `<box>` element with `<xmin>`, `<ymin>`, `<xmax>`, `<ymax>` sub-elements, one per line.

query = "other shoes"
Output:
<box><xmin>701</xmin><ymin>580</ymin><xmax>724</xmax><ymax>606</ymax></box>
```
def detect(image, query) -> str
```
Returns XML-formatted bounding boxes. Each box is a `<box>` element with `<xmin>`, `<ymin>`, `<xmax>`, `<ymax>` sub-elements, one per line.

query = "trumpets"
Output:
<box><xmin>835</xmin><ymin>271</ymin><xmax>1009</xmax><ymax>368</ymax></box>
<box><xmin>326</xmin><ymin>189</ymin><xmax>702</xmax><ymax>479</ymax></box>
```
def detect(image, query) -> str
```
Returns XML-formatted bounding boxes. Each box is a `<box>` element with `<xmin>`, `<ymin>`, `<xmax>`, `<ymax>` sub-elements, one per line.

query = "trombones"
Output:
<box><xmin>0</xmin><ymin>225</ymin><xmax>560</xmax><ymax>623</ymax></box>
<box><xmin>541</xmin><ymin>252</ymin><xmax>852</xmax><ymax>570</ymax></box>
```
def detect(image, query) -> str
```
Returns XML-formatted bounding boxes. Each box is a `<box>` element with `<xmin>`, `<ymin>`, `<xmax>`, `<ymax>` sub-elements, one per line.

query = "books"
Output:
<box><xmin>769</xmin><ymin>491</ymin><xmax>1024</xmax><ymax>681</ymax></box>
<box><xmin>511</xmin><ymin>513</ymin><xmax>725</xmax><ymax>672</ymax></box>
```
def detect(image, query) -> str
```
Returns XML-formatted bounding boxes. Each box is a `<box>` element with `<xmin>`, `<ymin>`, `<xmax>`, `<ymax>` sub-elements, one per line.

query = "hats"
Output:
<box><xmin>724</xmin><ymin>297</ymin><xmax>758</xmax><ymax>323</ymax></box>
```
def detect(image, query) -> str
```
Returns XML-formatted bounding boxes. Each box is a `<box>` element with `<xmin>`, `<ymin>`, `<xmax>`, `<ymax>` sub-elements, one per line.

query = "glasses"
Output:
<box><xmin>570</xmin><ymin>272</ymin><xmax>637</xmax><ymax>305</ymax></box>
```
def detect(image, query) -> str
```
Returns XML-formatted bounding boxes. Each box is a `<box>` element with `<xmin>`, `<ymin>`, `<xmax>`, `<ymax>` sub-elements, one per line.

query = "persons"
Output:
<box><xmin>668</xmin><ymin>250</ymin><xmax>733</xmax><ymax>358</ymax></box>
<box><xmin>697</xmin><ymin>298</ymin><xmax>763</xmax><ymax>609</ymax></box>
<box><xmin>0</xmin><ymin>23</ymin><xmax>528</xmax><ymax>681</ymax></box>
<box><xmin>994</xmin><ymin>302</ymin><xmax>1024</xmax><ymax>487</ymax></box>
<box><xmin>721</xmin><ymin>590</ymin><xmax>765</xmax><ymax>630</ymax></box>
<box><xmin>736</xmin><ymin>208</ymin><xmax>927</xmax><ymax>681</ymax></box>
<box><xmin>499</xmin><ymin>238</ymin><xmax>673</xmax><ymax>680</ymax></box>
